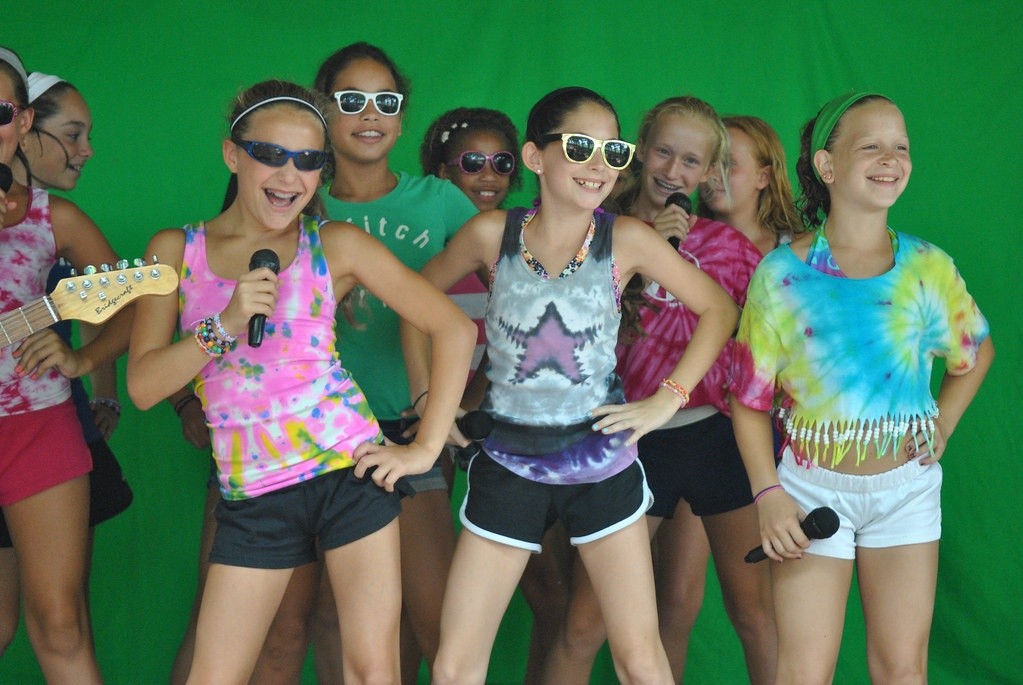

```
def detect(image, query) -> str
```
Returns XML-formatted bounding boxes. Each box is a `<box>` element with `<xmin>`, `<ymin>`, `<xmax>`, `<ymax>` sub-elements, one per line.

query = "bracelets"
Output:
<box><xmin>662</xmin><ymin>379</ymin><xmax>689</xmax><ymax>403</ymax></box>
<box><xmin>659</xmin><ymin>382</ymin><xmax>685</xmax><ymax>409</ymax></box>
<box><xmin>88</xmin><ymin>397</ymin><xmax>120</xmax><ymax>416</ymax></box>
<box><xmin>190</xmin><ymin>312</ymin><xmax>236</xmax><ymax>359</ymax></box>
<box><xmin>753</xmin><ymin>485</ymin><xmax>783</xmax><ymax>504</ymax></box>
<box><xmin>412</xmin><ymin>391</ymin><xmax>428</xmax><ymax>411</ymax></box>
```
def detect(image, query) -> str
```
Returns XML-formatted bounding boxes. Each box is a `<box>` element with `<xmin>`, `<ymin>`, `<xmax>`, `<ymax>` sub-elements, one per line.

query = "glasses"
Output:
<box><xmin>543</xmin><ymin>132</ymin><xmax>636</xmax><ymax>171</ymax></box>
<box><xmin>443</xmin><ymin>150</ymin><xmax>515</xmax><ymax>175</ymax></box>
<box><xmin>231</xmin><ymin>137</ymin><xmax>329</xmax><ymax>172</ymax></box>
<box><xmin>331</xmin><ymin>90</ymin><xmax>404</xmax><ymax>116</ymax></box>
<box><xmin>0</xmin><ymin>101</ymin><xmax>22</xmax><ymax>127</ymax></box>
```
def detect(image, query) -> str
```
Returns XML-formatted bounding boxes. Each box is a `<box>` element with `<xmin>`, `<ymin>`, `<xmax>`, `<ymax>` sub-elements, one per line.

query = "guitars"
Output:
<box><xmin>0</xmin><ymin>254</ymin><xmax>179</xmax><ymax>350</ymax></box>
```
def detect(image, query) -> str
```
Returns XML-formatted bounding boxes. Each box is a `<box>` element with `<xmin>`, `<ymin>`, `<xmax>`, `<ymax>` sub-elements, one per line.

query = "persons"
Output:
<box><xmin>650</xmin><ymin>117</ymin><xmax>807</xmax><ymax>685</ymax></box>
<box><xmin>127</xmin><ymin>80</ymin><xmax>478</xmax><ymax>685</ymax></box>
<box><xmin>0</xmin><ymin>71</ymin><xmax>121</xmax><ymax>658</ymax></box>
<box><xmin>540</xmin><ymin>96</ymin><xmax>780</xmax><ymax>685</ymax></box>
<box><xmin>728</xmin><ymin>94</ymin><xmax>994</xmax><ymax>685</ymax></box>
<box><xmin>609</xmin><ymin>153</ymin><xmax>642</xmax><ymax>198</ymax></box>
<box><xmin>399</xmin><ymin>105</ymin><xmax>571</xmax><ymax>685</ymax></box>
<box><xmin>247</xmin><ymin>41</ymin><xmax>480</xmax><ymax>685</ymax></box>
<box><xmin>400</xmin><ymin>85</ymin><xmax>737</xmax><ymax>685</ymax></box>
<box><xmin>165</xmin><ymin>173</ymin><xmax>344</xmax><ymax>685</ymax></box>
<box><xmin>0</xmin><ymin>46</ymin><xmax>136</xmax><ymax>685</ymax></box>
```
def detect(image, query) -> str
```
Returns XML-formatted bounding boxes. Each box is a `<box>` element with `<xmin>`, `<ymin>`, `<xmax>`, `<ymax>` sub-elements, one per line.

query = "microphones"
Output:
<box><xmin>665</xmin><ymin>192</ymin><xmax>692</xmax><ymax>252</ymax></box>
<box><xmin>744</xmin><ymin>506</ymin><xmax>840</xmax><ymax>564</ymax></box>
<box><xmin>455</xmin><ymin>411</ymin><xmax>494</xmax><ymax>441</ymax></box>
<box><xmin>248</xmin><ymin>249</ymin><xmax>280</xmax><ymax>348</ymax></box>
